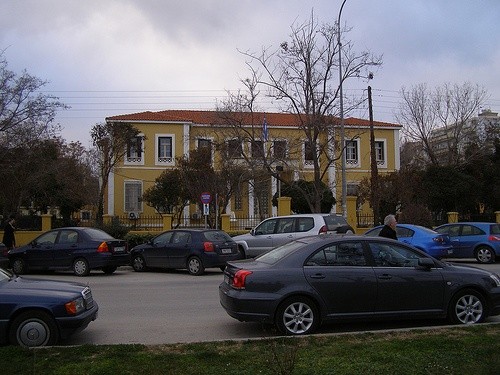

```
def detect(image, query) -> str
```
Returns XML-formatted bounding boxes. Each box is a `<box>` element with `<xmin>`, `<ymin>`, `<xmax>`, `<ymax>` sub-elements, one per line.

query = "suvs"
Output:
<box><xmin>232</xmin><ymin>213</ymin><xmax>361</xmax><ymax>263</ymax></box>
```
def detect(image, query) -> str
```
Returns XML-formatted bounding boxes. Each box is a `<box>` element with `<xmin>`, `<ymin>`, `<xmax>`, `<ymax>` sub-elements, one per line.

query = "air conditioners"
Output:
<box><xmin>192</xmin><ymin>212</ymin><xmax>201</xmax><ymax>219</ymax></box>
<box><xmin>128</xmin><ymin>211</ymin><xmax>140</xmax><ymax>220</ymax></box>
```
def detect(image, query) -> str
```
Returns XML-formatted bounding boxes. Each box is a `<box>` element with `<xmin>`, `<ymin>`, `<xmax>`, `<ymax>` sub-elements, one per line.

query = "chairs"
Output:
<box><xmin>70</xmin><ymin>235</ymin><xmax>78</xmax><ymax>243</ymax></box>
<box><xmin>180</xmin><ymin>234</ymin><xmax>189</xmax><ymax>243</ymax></box>
<box><xmin>341</xmin><ymin>247</ymin><xmax>362</xmax><ymax>266</ymax></box>
<box><xmin>284</xmin><ymin>224</ymin><xmax>292</xmax><ymax>233</ymax></box>
<box><xmin>59</xmin><ymin>234</ymin><xmax>68</xmax><ymax>244</ymax></box>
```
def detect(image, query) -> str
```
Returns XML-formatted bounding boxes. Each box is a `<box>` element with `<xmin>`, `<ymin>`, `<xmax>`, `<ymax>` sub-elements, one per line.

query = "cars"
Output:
<box><xmin>363</xmin><ymin>223</ymin><xmax>454</xmax><ymax>260</ymax></box>
<box><xmin>218</xmin><ymin>232</ymin><xmax>500</xmax><ymax>335</ymax></box>
<box><xmin>0</xmin><ymin>267</ymin><xmax>99</xmax><ymax>346</ymax></box>
<box><xmin>433</xmin><ymin>222</ymin><xmax>500</xmax><ymax>264</ymax></box>
<box><xmin>130</xmin><ymin>227</ymin><xmax>242</xmax><ymax>276</ymax></box>
<box><xmin>7</xmin><ymin>227</ymin><xmax>133</xmax><ymax>277</ymax></box>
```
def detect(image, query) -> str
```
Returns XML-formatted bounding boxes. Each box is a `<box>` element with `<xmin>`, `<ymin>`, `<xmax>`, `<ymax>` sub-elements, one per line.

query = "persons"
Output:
<box><xmin>378</xmin><ymin>215</ymin><xmax>398</xmax><ymax>266</ymax></box>
<box><xmin>2</xmin><ymin>216</ymin><xmax>15</xmax><ymax>270</ymax></box>
